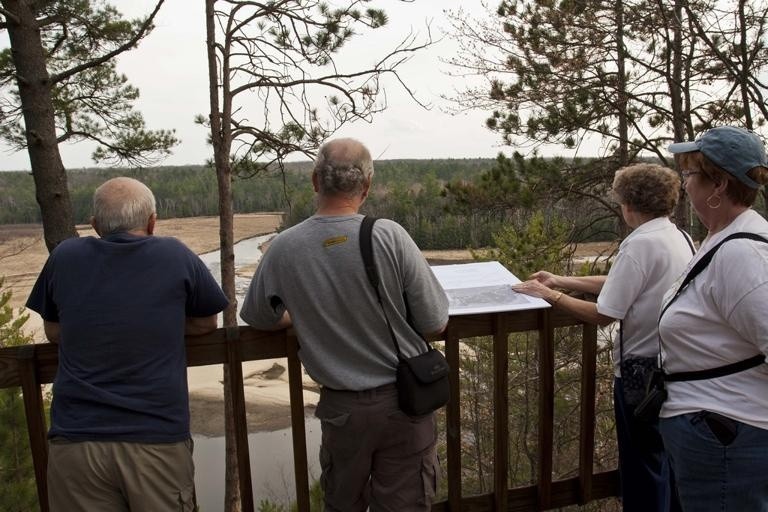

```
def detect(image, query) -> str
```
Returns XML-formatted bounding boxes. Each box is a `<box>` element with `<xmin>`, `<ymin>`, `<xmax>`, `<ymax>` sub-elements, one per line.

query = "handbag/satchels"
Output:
<box><xmin>394</xmin><ymin>348</ymin><xmax>452</xmax><ymax>416</ymax></box>
<box><xmin>633</xmin><ymin>367</ymin><xmax>669</xmax><ymax>422</ymax></box>
<box><xmin>620</xmin><ymin>356</ymin><xmax>658</xmax><ymax>406</ymax></box>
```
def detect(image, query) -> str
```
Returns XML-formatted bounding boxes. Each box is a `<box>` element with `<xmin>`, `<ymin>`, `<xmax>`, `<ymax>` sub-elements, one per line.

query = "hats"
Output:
<box><xmin>667</xmin><ymin>126</ymin><xmax>768</xmax><ymax>190</ymax></box>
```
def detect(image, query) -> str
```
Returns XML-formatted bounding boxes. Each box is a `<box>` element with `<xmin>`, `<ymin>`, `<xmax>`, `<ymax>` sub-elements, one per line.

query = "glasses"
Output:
<box><xmin>681</xmin><ymin>170</ymin><xmax>702</xmax><ymax>181</ymax></box>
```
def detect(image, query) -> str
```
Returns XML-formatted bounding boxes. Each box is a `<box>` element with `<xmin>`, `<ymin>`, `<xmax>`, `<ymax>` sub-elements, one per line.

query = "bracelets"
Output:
<box><xmin>553</xmin><ymin>292</ymin><xmax>563</xmax><ymax>306</ymax></box>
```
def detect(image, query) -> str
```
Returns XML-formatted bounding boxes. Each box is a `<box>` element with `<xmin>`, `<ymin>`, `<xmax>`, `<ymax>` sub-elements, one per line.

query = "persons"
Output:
<box><xmin>510</xmin><ymin>161</ymin><xmax>696</xmax><ymax>512</ymax></box>
<box><xmin>656</xmin><ymin>125</ymin><xmax>768</xmax><ymax>512</ymax></box>
<box><xmin>24</xmin><ymin>177</ymin><xmax>230</xmax><ymax>512</ymax></box>
<box><xmin>238</xmin><ymin>138</ymin><xmax>450</xmax><ymax>512</ymax></box>
